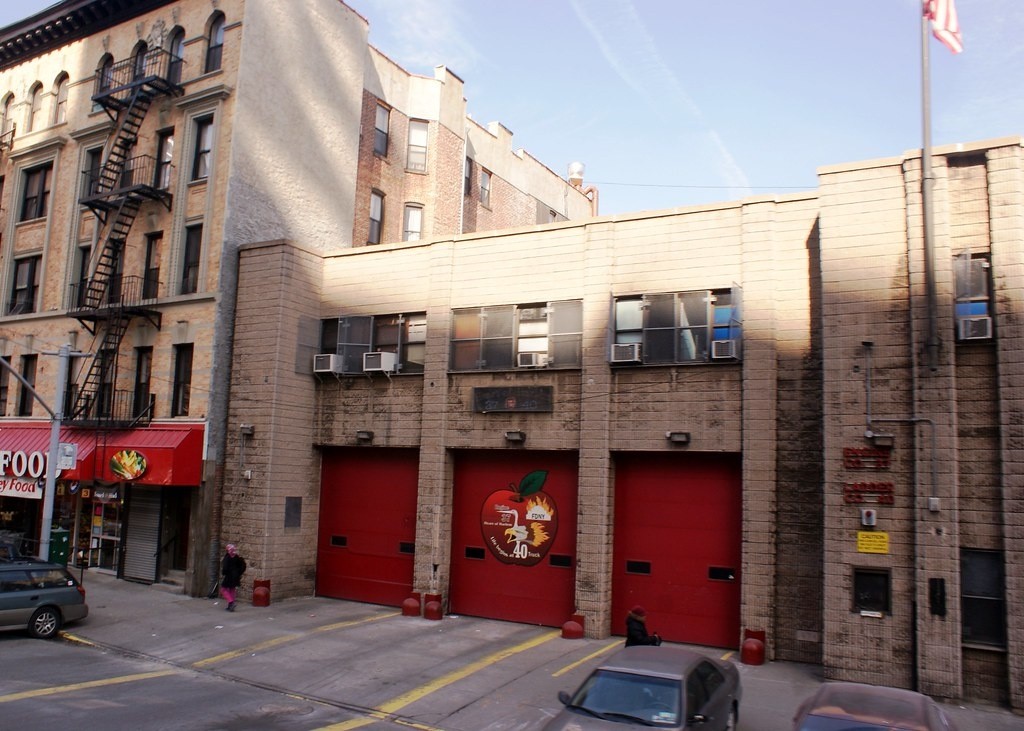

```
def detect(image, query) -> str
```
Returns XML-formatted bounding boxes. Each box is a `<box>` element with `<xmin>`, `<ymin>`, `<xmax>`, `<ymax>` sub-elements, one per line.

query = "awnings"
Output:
<box><xmin>0</xmin><ymin>422</ymin><xmax>109</xmax><ymax>481</ymax></box>
<box><xmin>95</xmin><ymin>427</ymin><xmax>204</xmax><ymax>486</ymax></box>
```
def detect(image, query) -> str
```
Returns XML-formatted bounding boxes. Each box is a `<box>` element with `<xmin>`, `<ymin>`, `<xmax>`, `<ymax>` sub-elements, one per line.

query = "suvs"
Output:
<box><xmin>0</xmin><ymin>560</ymin><xmax>89</xmax><ymax>640</ymax></box>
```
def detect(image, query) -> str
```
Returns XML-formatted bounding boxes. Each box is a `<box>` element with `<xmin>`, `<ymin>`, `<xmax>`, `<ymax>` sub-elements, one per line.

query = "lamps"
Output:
<box><xmin>357</xmin><ymin>431</ymin><xmax>374</xmax><ymax>439</ymax></box>
<box><xmin>671</xmin><ymin>432</ymin><xmax>690</xmax><ymax>443</ymax></box>
<box><xmin>506</xmin><ymin>432</ymin><xmax>526</xmax><ymax>441</ymax></box>
<box><xmin>874</xmin><ymin>433</ymin><xmax>894</xmax><ymax>446</ymax></box>
<box><xmin>241</xmin><ymin>424</ymin><xmax>258</xmax><ymax>433</ymax></box>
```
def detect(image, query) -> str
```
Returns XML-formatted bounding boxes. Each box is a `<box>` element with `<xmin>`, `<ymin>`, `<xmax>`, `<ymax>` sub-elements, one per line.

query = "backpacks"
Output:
<box><xmin>232</xmin><ymin>554</ymin><xmax>247</xmax><ymax>575</ymax></box>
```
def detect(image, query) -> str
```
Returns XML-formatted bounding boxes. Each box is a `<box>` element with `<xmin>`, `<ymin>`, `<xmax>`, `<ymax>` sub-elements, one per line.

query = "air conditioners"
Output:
<box><xmin>362</xmin><ymin>351</ymin><xmax>400</xmax><ymax>372</ymax></box>
<box><xmin>712</xmin><ymin>339</ymin><xmax>738</xmax><ymax>358</ymax></box>
<box><xmin>611</xmin><ymin>343</ymin><xmax>643</xmax><ymax>363</ymax></box>
<box><xmin>313</xmin><ymin>353</ymin><xmax>344</xmax><ymax>373</ymax></box>
<box><xmin>958</xmin><ymin>318</ymin><xmax>993</xmax><ymax>339</ymax></box>
<box><xmin>517</xmin><ymin>353</ymin><xmax>541</xmax><ymax>367</ymax></box>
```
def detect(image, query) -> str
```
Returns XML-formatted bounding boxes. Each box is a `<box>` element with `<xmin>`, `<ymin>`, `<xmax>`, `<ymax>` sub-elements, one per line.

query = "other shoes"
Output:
<box><xmin>225</xmin><ymin>601</ymin><xmax>236</xmax><ymax>612</ymax></box>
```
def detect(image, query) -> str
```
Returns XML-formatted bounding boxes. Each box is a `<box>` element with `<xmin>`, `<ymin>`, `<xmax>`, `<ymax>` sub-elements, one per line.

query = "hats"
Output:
<box><xmin>630</xmin><ymin>605</ymin><xmax>645</xmax><ymax>617</ymax></box>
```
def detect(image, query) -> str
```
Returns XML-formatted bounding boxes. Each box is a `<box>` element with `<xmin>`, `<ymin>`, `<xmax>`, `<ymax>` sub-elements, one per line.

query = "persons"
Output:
<box><xmin>219</xmin><ymin>544</ymin><xmax>246</xmax><ymax>612</ymax></box>
<box><xmin>625</xmin><ymin>606</ymin><xmax>658</xmax><ymax>649</ymax></box>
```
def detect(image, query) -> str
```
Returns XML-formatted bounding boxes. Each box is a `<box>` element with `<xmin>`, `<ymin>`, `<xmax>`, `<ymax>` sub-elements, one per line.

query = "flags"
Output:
<box><xmin>925</xmin><ymin>0</ymin><xmax>963</xmax><ymax>54</ymax></box>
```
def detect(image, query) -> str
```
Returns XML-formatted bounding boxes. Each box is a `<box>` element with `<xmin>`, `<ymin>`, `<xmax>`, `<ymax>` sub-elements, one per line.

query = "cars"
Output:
<box><xmin>541</xmin><ymin>645</ymin><xmax>742</xmax><ymax>730</ymax></box>
<box><xmin>791</xmin><ymin>682</ymin><xmax>958</xmax><ymax>731</ymax></box>
